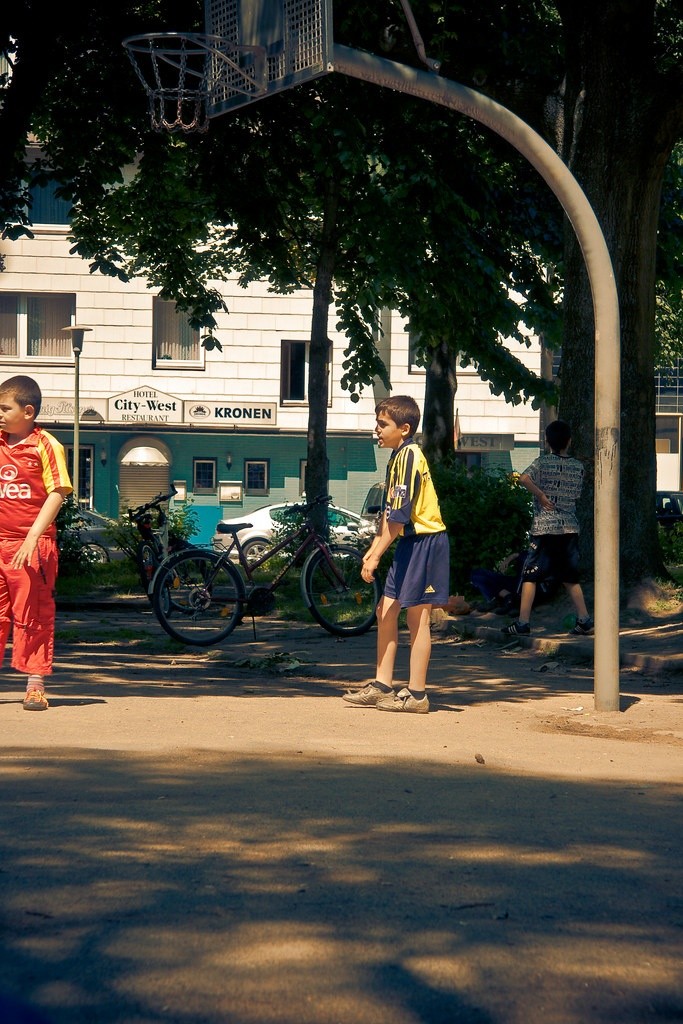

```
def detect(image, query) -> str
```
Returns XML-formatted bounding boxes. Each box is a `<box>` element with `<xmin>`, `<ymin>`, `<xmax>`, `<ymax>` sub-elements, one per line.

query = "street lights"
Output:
<box><xmin>62</xmin><ymin>324</ymin><xmax>94</xmax><ymax>509</ymax></box>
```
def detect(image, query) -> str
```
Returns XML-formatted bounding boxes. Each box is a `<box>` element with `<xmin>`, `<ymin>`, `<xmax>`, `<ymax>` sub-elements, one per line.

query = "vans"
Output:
<box><xmin>360</xmin><ymin>482</ymin><xmax>387</xmax><ymax>525</ymax></box>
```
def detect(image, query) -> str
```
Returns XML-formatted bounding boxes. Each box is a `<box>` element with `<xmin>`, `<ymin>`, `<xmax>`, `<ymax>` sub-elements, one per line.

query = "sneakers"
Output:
<box><xmin>567</xmin><ymin>617</ymin><xmax>594</xmax><ymax>634</ymax></box>
<box><xmin>501</xmin><ymin>620</ymin><xmax>531</xmax><ymax>636</ymax></box>
<box><xmin>376</xmin><ymin>687</ymin><xmax>429</xmax><ymax>714</ymax></box>
<box><xmin>23</xmin><ymin>686</ymin><xmax>49</xmax><ymax>710</ymax></box>
<box><xmin>342</xmin><ymin>683</ymin><xmax>398</xmax><ymax>705</ymax></box>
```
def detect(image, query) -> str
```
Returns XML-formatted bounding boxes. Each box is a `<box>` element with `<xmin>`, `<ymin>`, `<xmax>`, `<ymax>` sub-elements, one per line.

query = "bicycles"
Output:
<box><xmin>127</xmin><ymin>486</ymin><xmax>216</xmax><ymax>617</ymax></box>
<box><xmin>147</xmin><ymin>493</ymin><xmax>381</xmax><ymax>646</ymax></box>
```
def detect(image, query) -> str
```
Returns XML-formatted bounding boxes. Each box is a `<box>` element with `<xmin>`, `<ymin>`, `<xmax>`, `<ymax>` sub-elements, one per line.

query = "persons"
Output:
<box><xmin>500</xmin><ymin>420</ymin><xmax>595</xmax><ymax>636</ymax></box>
<box><xmin>0</xmin><ymin>376</ymin><xmax>75</xmax><ymax>711</ymax></box>
<box><xmin>343</xmin><ymin>395</ymin><xmax>450</xmax><ymax>713</ymax></box>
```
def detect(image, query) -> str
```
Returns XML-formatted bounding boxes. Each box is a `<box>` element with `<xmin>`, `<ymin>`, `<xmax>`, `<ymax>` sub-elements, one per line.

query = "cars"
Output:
<box><xmin>212</xmin><ymin>500</ymin><xmax>377</xmax><ymax>566</ymax></box>
<box><xmin>656</xmin><ymin>491</ymin><xmax>683</xmax><ymax>536</ymax></box>
<box><xmin>56</xmin><ymin>508</ymin><xmax>132</xmax><ymax>575</ymax></box>
